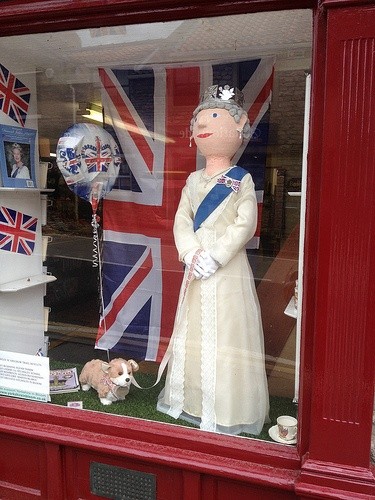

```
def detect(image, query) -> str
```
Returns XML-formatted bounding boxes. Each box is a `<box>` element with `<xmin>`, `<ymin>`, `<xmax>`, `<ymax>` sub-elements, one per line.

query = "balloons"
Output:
<box><xmin>58</xmin><ymin>123</ymin><xmax>121</xmax><ymax>214</ymax></box>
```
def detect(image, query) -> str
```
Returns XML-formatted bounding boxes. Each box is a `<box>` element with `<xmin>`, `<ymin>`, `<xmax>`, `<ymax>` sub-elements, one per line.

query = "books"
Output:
<box><xmin>49</xmin><ymin>368</ymin><xmax>80</xmax><ymax>394</ymax></box>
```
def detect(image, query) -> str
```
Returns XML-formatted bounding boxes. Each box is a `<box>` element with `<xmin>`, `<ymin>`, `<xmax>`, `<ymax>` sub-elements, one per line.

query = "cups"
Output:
<box><xmin>276</xmin><ymin>415</ymin><xmax>298</xmax><ymax>440</ymax></box>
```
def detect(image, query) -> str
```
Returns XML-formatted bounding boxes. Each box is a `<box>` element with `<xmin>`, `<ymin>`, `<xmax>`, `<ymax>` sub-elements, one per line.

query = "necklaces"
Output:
<box><xmin>201</xmin><ymin>166</ymin><xmax>230</xmax><ymax>187</ymax></box>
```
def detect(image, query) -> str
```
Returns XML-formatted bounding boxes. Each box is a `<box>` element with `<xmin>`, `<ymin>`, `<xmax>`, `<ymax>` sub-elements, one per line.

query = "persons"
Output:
<box><xmin>10</xmin><ymin>144</ymin><xmax>30</xmax><ymax>179</ymax></box>
<box><xmin>157</xmin><ymin>85</ymin><xmax>271</xmax><ymax>434</ymax></box>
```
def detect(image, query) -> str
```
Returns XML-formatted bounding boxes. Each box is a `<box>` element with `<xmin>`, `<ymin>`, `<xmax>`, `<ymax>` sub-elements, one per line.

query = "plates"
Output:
<box><xmin>268</xmin><ymin>424</ymin><xmax>297</xmax><ymax>444</ymax></box>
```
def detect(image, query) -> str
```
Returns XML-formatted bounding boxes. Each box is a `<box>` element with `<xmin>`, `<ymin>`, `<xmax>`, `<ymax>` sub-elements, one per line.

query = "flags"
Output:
<box><xmin>94</xmin><ymin>54</ymin><xmax>276</xmax><ymax>367</ymax></box>
<box><xmin>0</xmin><ymin>64</ymin><xmax>31</xmax><ymax>127</ymax></box>
<box><xmin>0</xmin><ymin>206</ymin><xmax>38</xmax><ymax>256</ymax></box>
